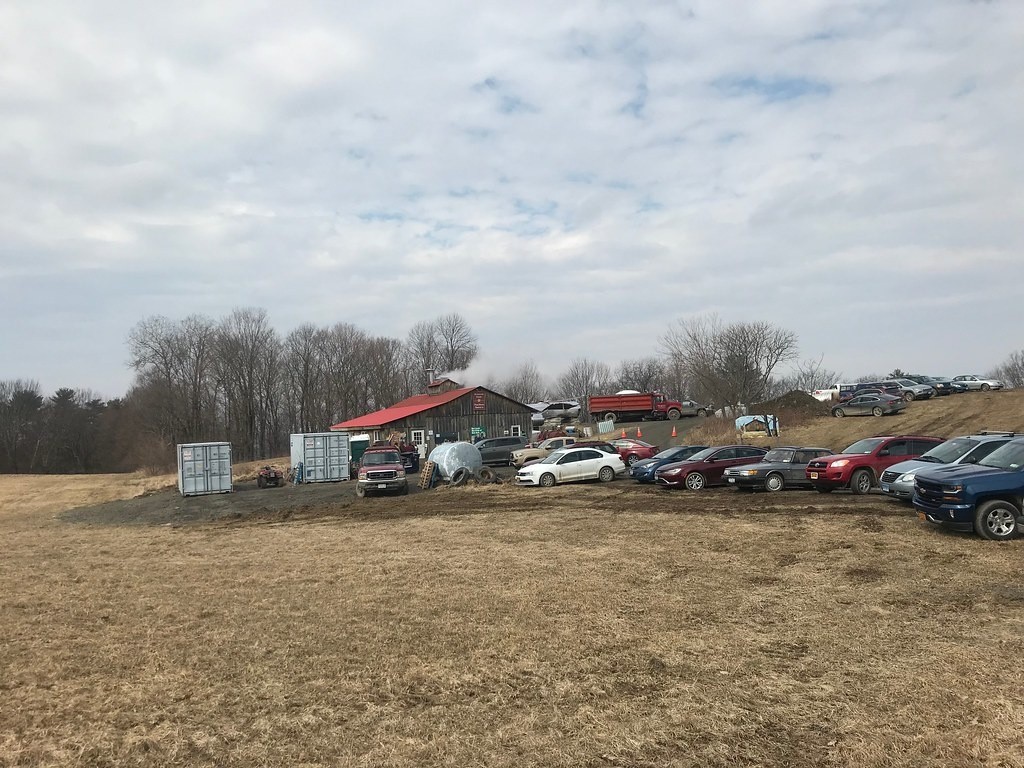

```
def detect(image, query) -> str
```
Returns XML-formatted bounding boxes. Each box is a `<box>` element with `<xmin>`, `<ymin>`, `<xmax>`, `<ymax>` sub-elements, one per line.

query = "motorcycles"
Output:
<box><xmin>257</xmin><ymin>465</ymin><xmax>284</xmax><ymax>489</ymax></box>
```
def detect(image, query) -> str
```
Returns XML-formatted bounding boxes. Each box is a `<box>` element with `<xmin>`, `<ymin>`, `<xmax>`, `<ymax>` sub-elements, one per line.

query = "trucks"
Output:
<box><xmin>588</xmin><ymin>390</ymin><xmax>682</xmax><ymax>422</ymax></box>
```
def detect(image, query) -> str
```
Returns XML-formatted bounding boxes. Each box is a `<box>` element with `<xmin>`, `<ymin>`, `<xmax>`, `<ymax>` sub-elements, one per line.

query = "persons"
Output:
<box><xmin>393</xmin><ymin>440</ymin><xmax>419</xmax><ymax>454</ymax></box>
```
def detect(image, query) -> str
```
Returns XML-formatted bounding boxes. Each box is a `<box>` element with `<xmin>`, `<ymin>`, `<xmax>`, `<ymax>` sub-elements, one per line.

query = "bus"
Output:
<box><xmin>830</xmin><ymin>383</ymin><xmax>857</xmax><ymax>395</ymax></box>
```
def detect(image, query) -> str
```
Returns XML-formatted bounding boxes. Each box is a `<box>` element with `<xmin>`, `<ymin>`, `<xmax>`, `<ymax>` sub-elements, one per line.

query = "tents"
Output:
<box><xmin>736</xmin><ymin>413</ymin><xmax>780</xmax><ymax>438</ymax></box>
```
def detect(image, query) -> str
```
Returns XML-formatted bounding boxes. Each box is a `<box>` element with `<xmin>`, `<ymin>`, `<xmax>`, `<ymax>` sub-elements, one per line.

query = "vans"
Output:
<box><xmin>519</xmin><ymin>440</ymin><xmax>620</xmax><ymax>471</ymax></box>
<box><xmin>472</xmin><ymin>436</ymin><xmax>529</xmax><ymax>467</ymax></box>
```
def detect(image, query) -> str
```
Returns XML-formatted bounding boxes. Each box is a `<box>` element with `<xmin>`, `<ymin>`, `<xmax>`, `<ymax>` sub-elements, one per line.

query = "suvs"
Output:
<box><xmin>883</xmin><ymin>378</ymin><xmax>934</xmax><ymax>401</ymax></box>
<box><xmin>912</xmin><ymin>376</ymin><xmax>952</xmax><ymax>397</ymax></box>
<box><xmin>804</xmin><ymin>436</ymin><xmax>948</xmax><ymax>495</ymax></box>
<box><xmin>879</xmin><ymin>430</ymin><xmax>1024</xmax><ymax>502</ymax></box>
<box><xmin>355</xmin><ymin>446</ymin><xmax>409</xmax><ymax>499</ymax></box>
<box><xmin>680</xmin><ymin>399</ymin><xmax>715</xmax><ymax>419</ymax></box>
<box><xmin>857</xmin><ymin>383</ymin><xmax>905</xmax><ymax>399</ymax></box>
<box><xmin>398</xmin><ymin>441</ymin><xmax>421</xmax><ymax>474</ymax></box>
<box><xmin>912</xmin><ymin>437</ymin><xmax>1024</xmax><ymax>540</ymax></box>
<box><xmin>541</xmin><ymin>401</ymin><xmax>581</xmax><ymax>420</ymax></box>
<box><xmin>509</xmin><ymin>436</ymin><xmax>578</xmax><ymax>470</ymax></box>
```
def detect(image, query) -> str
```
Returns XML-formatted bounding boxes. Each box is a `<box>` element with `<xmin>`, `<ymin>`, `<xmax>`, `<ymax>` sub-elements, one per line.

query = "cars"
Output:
<box><xmin>557</xmin><ymin>427</ymin><xmax>589</xmax><ymax>440</ymax></box>
<box><xmin>515</xmin><ymin>448</ymin><xmax>626</xmax><ymax>488</ymax></box>
<box><xmin>840</xmin><ymin>388</ymin><xmax>894</xmax><ymax>400</ymax></box>
<box><xmin>721</xmin><ymin>446</ymin><xmax>839</xmax><ymax>493</ymax></box>
<box><xmin>628</xmin><ymin>445</ymin><xmax>711</xmax><ymax>484</ymax></box>
<box><xmin>934</xmin><ymin>376</ymin><xmax>971</xmax><ymax>394</ymax></box>
<box><xmin>609</xmin><ymin>439</ymin><xmax>660</xmax><ymax>468</ymax></box>
<box><xmin>831</xmin><ymin>393</ymin><xmax>905</xmax><ymax>417</ymax></box>
<box><xmin>654</xmin><ymin>445</ymin><xmax>774</xmax><ymax>491</ymax></box>
<box><xmin>714</xmin><ymin>404</ymin><xmax>755</xmax><ymax>418</ymax></box>
<box><xmin>952</xmin><ymin>375</ymin><xmax>1005</xmax><ymax>392</ymax></box>
